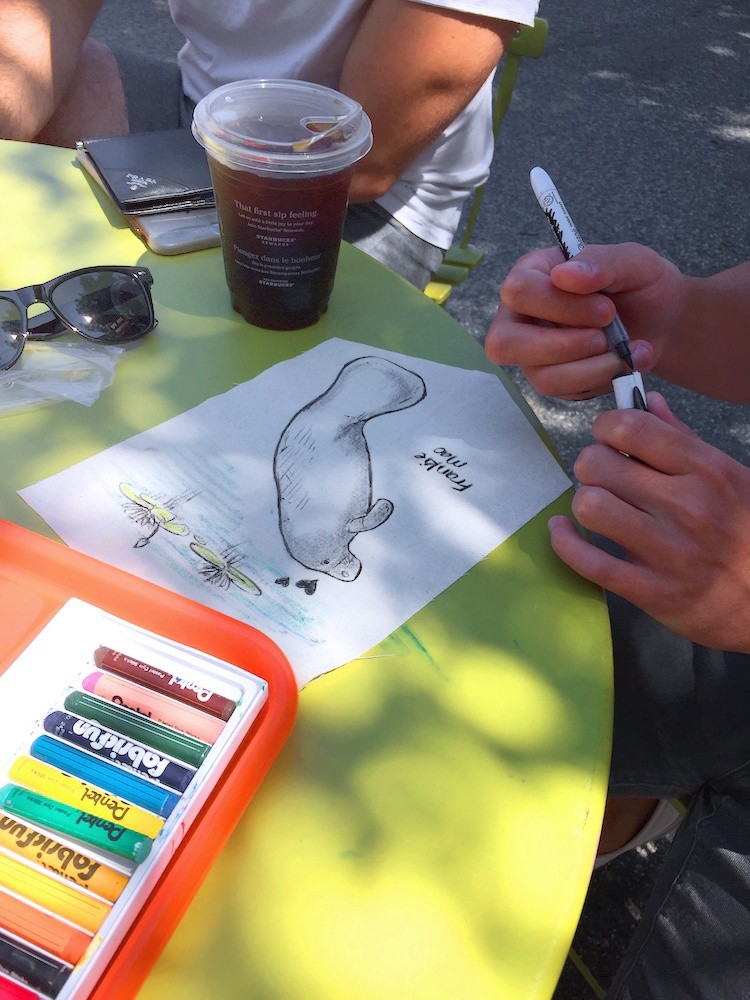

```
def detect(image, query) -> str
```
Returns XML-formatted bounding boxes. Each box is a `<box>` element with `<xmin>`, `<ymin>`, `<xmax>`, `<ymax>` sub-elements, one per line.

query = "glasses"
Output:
<box><xmin>1</xmin><ymin>266</ymin><xmax>157</xmax><ymax>371</ymax></box>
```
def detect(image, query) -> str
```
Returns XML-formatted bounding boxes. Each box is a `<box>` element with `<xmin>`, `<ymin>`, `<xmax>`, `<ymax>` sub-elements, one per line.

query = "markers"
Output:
<box><xmin>1</xmin><ymin>644</ymin><xmax>241</xmax><ymax>1000</ymax></box>
<box><xmin>529</xmin><ymin>164</ymin><xmax>639</xmax><ymax>370</ymax></box>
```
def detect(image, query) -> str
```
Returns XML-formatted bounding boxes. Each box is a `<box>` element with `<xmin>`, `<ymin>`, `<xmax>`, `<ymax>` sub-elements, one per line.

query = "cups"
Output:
<box><xmin>192</xmin><ymin>77</ymin><xmax>372</xmax><ymax>332</ymax></box>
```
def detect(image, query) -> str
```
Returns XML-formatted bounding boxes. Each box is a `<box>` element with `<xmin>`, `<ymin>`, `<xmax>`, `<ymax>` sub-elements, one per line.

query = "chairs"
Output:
<box><xmin>424</xmin><ymin>17</ymin><xmax>550</xmax><ymax>305</ymax></box>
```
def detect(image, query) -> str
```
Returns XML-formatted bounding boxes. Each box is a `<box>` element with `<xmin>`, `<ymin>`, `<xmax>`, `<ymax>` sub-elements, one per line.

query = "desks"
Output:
<box><xmin>0</xmin><ymin>137</ymin><xmax>613</xmax><ymax>1000</ymax></box>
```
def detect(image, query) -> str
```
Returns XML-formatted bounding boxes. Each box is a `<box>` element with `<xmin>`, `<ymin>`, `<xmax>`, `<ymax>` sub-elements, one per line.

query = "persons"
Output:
<box><xmin>484</xmin><ymin>242</ymin><xmax>749</xmax><ymax>1000</ymax></box>
<box><xmin>0</xmin><ymin>1</ymin><xmax>542</xmax><ymax>294</ymax></box>
